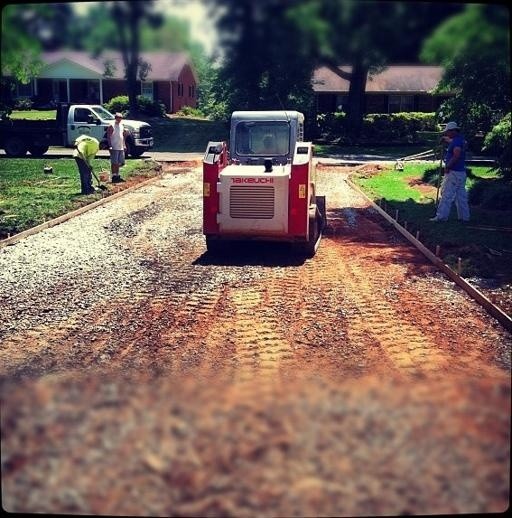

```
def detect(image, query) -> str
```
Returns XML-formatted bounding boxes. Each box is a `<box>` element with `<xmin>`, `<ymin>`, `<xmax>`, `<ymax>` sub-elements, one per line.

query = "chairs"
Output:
<box><xmin>430</xmin><ymin>216</ymin><xmax>448</xmax><ymax>223</ymax></box>
<box><xmin>112</xmin><ymin>175</ymin><xmax>126</xmax><ymax>183</ymax></box>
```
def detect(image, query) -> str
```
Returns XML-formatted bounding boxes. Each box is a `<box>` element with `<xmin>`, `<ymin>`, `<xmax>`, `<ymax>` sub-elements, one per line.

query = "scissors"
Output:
<box><xmin>75</xmin><ymin>145</ymin><xmax>107</xmax><ymax>191</ymax></box>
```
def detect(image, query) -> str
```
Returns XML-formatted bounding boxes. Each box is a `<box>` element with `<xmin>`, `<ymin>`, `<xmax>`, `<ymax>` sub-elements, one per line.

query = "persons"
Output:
<box><xmin>428</xmin><ymin>121</ymin><xmax>471</xmax><ymax>223</ymax></box>
<box><xmin>256</xmin><ymin>134</ymin><xmax>277</xmax><ymax>153</ymax></box>
<box><xmin>73</xmin><ymin>134</ymin><xmax>109</xmax><ymax>195</ymax></box>
<box><xmin>107</xmin><ymin>113</ymin><xmax>127</xmax><ymax>183</ymax></box>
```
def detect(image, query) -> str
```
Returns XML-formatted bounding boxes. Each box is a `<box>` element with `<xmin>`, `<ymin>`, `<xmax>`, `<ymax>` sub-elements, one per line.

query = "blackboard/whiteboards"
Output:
<box><xmin>199</xmin><ymin>108</ymin><xmax>329</xmax><ymax>260</ymax></box>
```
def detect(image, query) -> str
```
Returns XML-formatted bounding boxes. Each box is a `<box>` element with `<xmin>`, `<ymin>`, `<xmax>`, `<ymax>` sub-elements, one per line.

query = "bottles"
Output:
<box><xmin>116</xmin><ymin>113</ymin><xmax>123</xmax><ymax>118</ymax></box>
<box><xmin>442</xmin><ymin>122</ymin><xmax>461</xmax><ymax>132</ymax></box>
